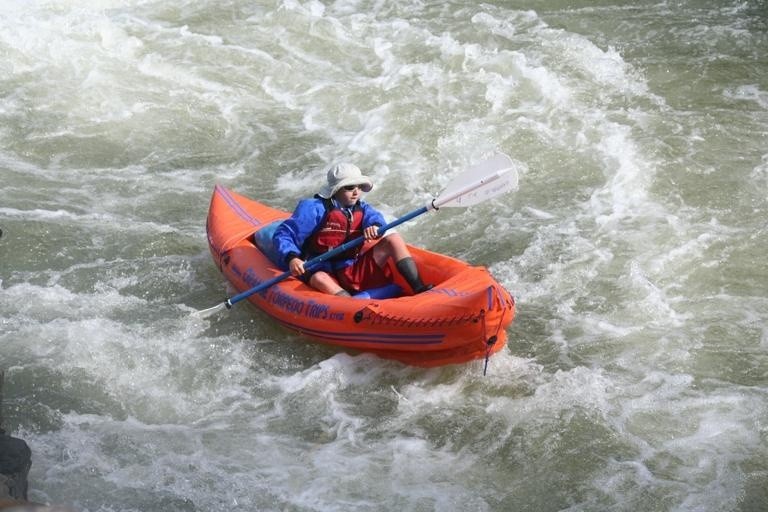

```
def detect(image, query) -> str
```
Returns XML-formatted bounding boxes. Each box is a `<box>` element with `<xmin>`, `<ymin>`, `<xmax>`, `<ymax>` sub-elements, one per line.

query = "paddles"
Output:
<box><xmin>190</xmin><ymin>153</ymin><xmax>518</xmax><ymax>318</ymax></box>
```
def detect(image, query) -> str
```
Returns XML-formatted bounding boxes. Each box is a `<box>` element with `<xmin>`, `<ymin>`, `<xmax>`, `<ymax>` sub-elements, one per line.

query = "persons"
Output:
<box><xmin>272</xmin><ymin>161</ymin><xmax>433</xmax><ymax>297</ymax></box>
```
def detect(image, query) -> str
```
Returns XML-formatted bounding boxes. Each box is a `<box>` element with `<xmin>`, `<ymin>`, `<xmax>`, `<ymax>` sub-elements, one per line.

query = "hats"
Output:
<box><xmin>313</xmin><ymin>163</ymin><xmax>373</xmax><ymax>200</ymax></box>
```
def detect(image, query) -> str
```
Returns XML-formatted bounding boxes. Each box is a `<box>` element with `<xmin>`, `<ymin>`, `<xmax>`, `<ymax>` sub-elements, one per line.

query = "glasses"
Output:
<box><xmin>339</xmin><ymin>184</ymin><xmax>363</xmax><ymax>193</ymax></box>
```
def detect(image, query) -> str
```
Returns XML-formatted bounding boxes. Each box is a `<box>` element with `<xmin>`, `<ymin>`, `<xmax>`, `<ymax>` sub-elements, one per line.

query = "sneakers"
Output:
<box><xmin>418</xmin><ymin>283</ymin><xmax>433</xmax><ymax>293</ymax></box>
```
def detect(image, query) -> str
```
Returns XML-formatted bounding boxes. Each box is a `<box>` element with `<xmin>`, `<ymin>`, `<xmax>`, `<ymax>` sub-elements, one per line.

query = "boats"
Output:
<box><xmin>205</xmin><ymin>182</ymin><xmax>516</xmax><ymax>368</ymax></box>
